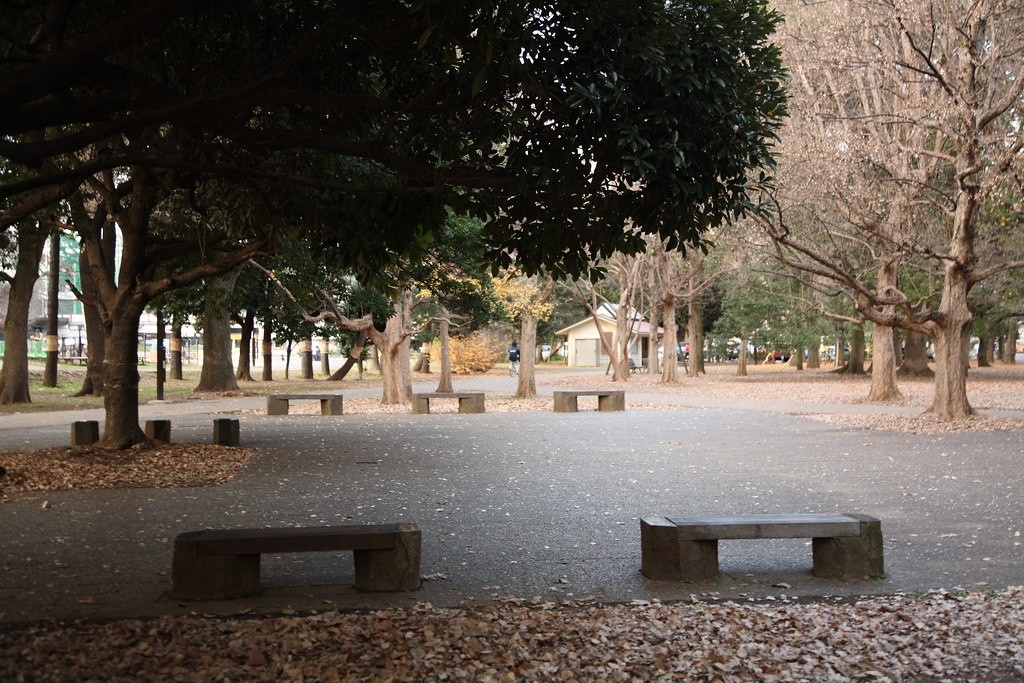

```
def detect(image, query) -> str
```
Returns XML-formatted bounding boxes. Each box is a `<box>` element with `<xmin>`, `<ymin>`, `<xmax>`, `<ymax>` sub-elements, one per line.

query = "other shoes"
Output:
<box><xmin>517</xmin><ymin>373</ymin><xmax>519</xmax><ymax>376</ymax></box>
<box><xmin>510</xmin><ymin>374</ymin><xmax>512</xmax><ymax>377</ymax></box>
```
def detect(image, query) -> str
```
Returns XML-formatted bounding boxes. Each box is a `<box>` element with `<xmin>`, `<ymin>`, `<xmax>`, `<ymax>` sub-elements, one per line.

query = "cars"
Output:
<box><xmin>726</xmin><ymin>345</ymin><xmax>739</xmax><ymax>359</ymax></box>
<box><xmin>824</xmin><ymin>347</ymin><xmax>849</xmax><ymax>362</ymax></box>
<box><xmin>678</xmin><ymin>342</ymin><xmax>689</xmax><ymax>358</ymax></box>
<box><xmin>1016</xmin><ymin>341</ymin><xmax>1024</xmax><ymax>353</ymax></box>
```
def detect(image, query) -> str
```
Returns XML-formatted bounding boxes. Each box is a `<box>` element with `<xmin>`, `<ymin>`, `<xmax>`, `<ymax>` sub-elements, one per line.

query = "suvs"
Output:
<box><xmin>755</xmin><ymin>343</ymin><xmax>791</xmax><ymax>362</ymax></box>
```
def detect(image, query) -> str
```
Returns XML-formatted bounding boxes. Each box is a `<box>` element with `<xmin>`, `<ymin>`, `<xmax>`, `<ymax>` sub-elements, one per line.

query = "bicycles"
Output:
<box><xmin>181</xmin><ymin>351</ymin><xmax>196</xmax><ymax>364</ymax></box>
<box><xmin>508</xmin><ymin>358</ymin><xmax>520</xmax><ymax>377</ymax></box>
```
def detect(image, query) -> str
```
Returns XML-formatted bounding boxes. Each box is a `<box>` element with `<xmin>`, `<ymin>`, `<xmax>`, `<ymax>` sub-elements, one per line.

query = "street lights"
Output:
<box><xmin>77</xmin><ymin>325</ymin><xmax>83</xmax><ymax>365</ymax></box>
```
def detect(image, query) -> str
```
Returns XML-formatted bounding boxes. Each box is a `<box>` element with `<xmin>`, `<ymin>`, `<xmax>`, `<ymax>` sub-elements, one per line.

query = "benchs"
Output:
<box><xmin>174</xmin><ymin>524</ymin><xmax>421</xmax><ymax>602</ymax></box>
<box><xmin>267</xmin><ymin>394</ymin><xmax>344</xmax><ymax>415</ymax></box>
<box><xmin>410</xmin><ymin>393</ymin><xmax>486</xmax><ymax>416</ymax></box>
<box><xmin>553</xmin><ymin>391</ymin><xmax>626</xmax><ymax>412</ymax></box>
<box><xmin>640</xmin><ymin>511</ymin><xmax>887</xmax><ymax>582</ymax></box>
<box><xmin>629</xmin><ymin>366</ymin><xmax>645</xmax><ymax>372</ymax></box>
<box><xmin>61</xmin><ymin>356</ymin><xmax>88</xmax><ymax>364</ymax></box>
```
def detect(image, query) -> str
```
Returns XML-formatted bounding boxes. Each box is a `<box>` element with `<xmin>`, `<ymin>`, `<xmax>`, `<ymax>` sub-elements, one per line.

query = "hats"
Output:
<box><xmin>512</xmin><ymin>342</ymin><xmax>517</xmax><ymax>345</ymax></box>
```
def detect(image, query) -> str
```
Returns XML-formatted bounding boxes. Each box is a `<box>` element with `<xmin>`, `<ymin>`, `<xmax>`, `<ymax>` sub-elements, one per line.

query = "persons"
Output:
<box><xmin>312</xmin><ymin>346</ymin><xmax>320</xmax><ymax>361</ymax></box>
<box><xmin>901</xmin><ymin>348</ymin><xmax>906</xmax><ymax>361</ymax></box>
<box><xmin>32</xmin><ymin>329</ymin><xmax>43</xmax><ymax>352</ymax></box>
<box><xmin>683</xmin><ymin>343</ymin><xmax>690</xmax><ymax>358</ymax></box>
<box><xmin>507</xmin><ymin>342</ymin><xmax>520</xmax><ymax>375</ymax></box>
<box><xmin>926</xmin><ymin>346</ymin><xmax>934</xmax><ymax>362</ymax></box>
